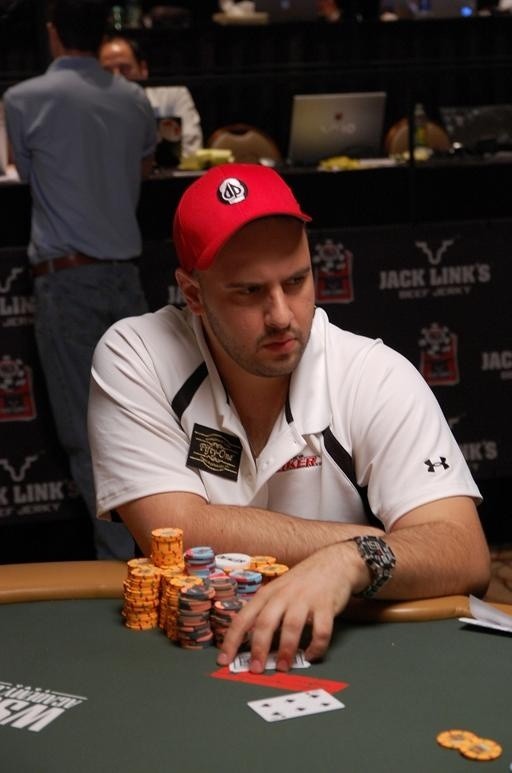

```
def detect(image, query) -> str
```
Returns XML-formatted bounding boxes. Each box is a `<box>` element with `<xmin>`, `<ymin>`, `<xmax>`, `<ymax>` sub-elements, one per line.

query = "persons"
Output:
<box><xmin>96</xmin><ymin>30</ymin><xmax>206</xmax><ymax>170</ymax></box>
<box><xmin>3</xmin><ymin>0</ymin><xmax>161</xmax><ymax>566</ymax></box>
<box><xmin>83</xmin><ymin>160</ymin><xmax>496</xmax><ymax>676</ymax></box>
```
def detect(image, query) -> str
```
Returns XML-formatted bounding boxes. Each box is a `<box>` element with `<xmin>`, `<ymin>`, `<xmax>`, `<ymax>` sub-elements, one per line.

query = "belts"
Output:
<box><xmin>28</xmin><ymin>250</ymin><xmax>133</xmax><ymax>279</ymax></box>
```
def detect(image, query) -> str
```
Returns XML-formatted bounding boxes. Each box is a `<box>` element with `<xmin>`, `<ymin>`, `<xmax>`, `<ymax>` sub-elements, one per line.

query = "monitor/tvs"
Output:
<box><xmin>287</xmin><ymin>90</ymin><xmax>388</xmax><ymax>165</ymax></box>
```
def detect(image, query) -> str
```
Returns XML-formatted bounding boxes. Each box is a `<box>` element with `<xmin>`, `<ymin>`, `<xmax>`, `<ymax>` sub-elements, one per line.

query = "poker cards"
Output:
<box><xmin>248</xmin><ymin>687</ymin><xmax>344</xmax><ymax>723</ymax></box>
<box><xmin>228</xmin><ymin>647</ymin><xmax>311</xmax><ymax>674</ymax></box>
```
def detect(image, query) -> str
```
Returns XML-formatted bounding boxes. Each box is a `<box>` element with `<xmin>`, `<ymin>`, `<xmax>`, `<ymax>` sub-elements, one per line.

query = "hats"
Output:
<box><xmin>170</xmin><ymin>162</ymin><xmax>316</xmax><ymax>275</ymax></box>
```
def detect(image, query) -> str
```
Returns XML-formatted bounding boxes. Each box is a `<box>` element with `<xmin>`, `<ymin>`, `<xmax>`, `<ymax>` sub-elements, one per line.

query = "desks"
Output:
<box><xmin>0</xmin><ymin>558</ymin><xmax>512</xmax><ymax>773</ymax></box>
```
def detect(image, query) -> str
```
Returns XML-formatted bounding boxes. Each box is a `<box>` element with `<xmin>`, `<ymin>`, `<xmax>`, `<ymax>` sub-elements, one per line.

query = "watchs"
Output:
<box><xmin>348</xmin><ymin>531</ymin><xmax>397</xmax><ymax>601</ymax></box>
